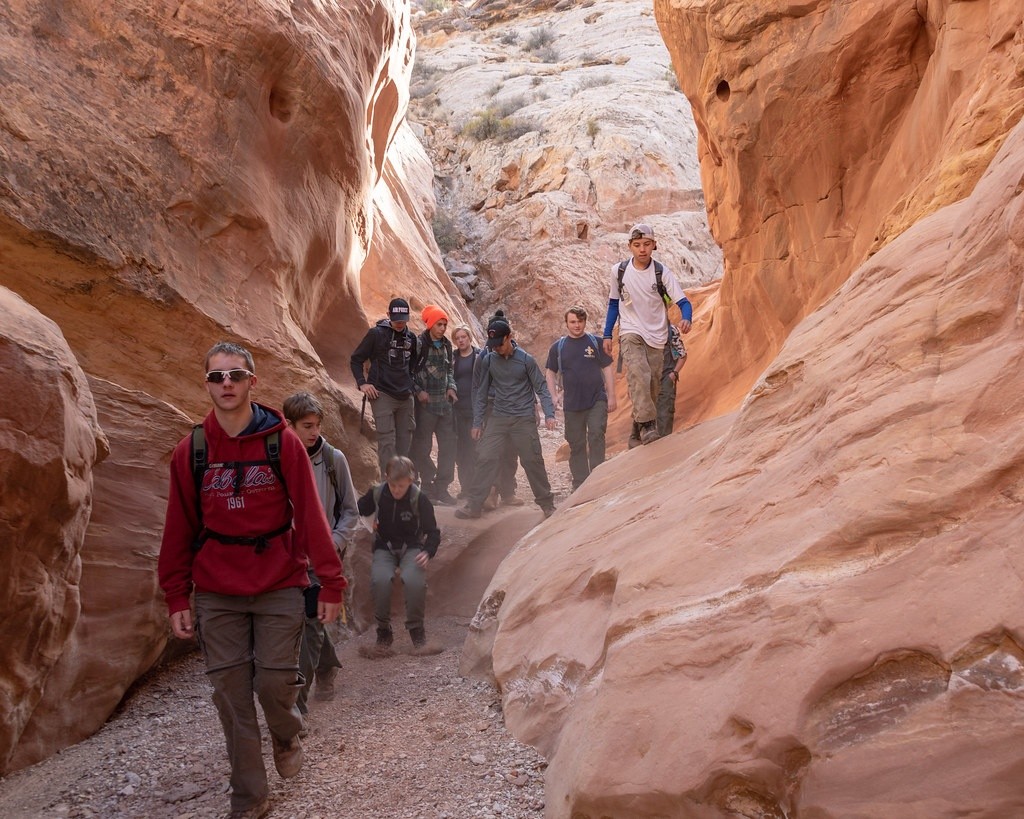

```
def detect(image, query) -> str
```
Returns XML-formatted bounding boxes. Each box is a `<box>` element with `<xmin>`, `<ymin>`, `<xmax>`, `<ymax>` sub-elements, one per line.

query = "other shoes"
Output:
<box><xmin>433</xmin><ymin>478</ymin><xmax>457</xmax><ymax>504</ymax></box>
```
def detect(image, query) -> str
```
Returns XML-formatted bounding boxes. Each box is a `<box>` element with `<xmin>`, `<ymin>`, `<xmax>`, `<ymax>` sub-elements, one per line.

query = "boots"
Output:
<box><xmin>409</xmin><ymin>627</ymin><xmax>428</xmax><ymax>654</ymax></box>
<box><xmin>629</xmin><ymin>420</ymin><xmax>644</xmax><ymax>450</ymax></box>
<box><xmin>535</xmin><ymin>492</ymin><xmax>557</xmax><ymax>517</ymax></box>
<box><xmin>482</xmin><ymin>486</ymin><xmax>498</xmax><ymax>510</ymax></box>
<box><xmin>376</xmin><ymin>624</ymin><xmax>393</xmax><ymax>661</ymax></box>
<box><xmin>638</xmin><ymin>419</ymin><xmax>662</xmax><ymax>446</ymax></box>
<box><xmin>454</xmin><ymin>500</ymin><xmax>481</xmax><ymax>519</ymax></box>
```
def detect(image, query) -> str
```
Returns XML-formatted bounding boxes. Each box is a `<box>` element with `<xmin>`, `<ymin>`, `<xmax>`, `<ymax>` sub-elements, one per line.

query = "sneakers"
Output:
<box><xmin>500</xmin><ymin>495</ymin><xmax>524</xmax><ymax>505</ymax></box>
<box><xmin>223</xmin><ymin>799</ymin><xmax>270</xmax><ymax>819</ymax></box>
<box><xmin>273</xmin><ymin>734</ymin><xmax>304</xmax><ymax>779</ymax></box>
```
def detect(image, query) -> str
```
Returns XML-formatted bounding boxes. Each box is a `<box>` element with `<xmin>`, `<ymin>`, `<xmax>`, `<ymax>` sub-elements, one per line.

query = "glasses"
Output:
<box><xmin>204</xmin><ymin>369</ymin><xmax>254</xmax><ymax>384</ymax></box>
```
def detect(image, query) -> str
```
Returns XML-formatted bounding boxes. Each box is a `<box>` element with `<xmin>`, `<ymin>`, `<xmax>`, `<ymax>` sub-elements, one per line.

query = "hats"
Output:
<box><xmin>389</xmin><ymin>298</ymin><xmax>409</xmax><ymax>322</ymax></box>
<box><xmin>628</xmin><ymin>224</ymin><xmax>657</xmax><ymax>250</ymax></box>
<box><xmin>422</xmin><ymin>305</ymin><xmax>448</xmax><ymax>330</ymax></box>
<box><xmin>486</xmin><ymin>321</ymin><xmax>511</xmax><ymax>346</ymax></box>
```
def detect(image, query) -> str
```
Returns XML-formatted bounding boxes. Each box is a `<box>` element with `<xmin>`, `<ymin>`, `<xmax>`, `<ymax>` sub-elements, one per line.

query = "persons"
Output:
<box><xmin>285</xmin><ymin>224</ymin><xmax>692</xmax><ymax>712</ymax></box>
<box><xmin>159</xmin><ymin>344</ymin><xmax>348</xmax><ymax>816</ymax></box>
